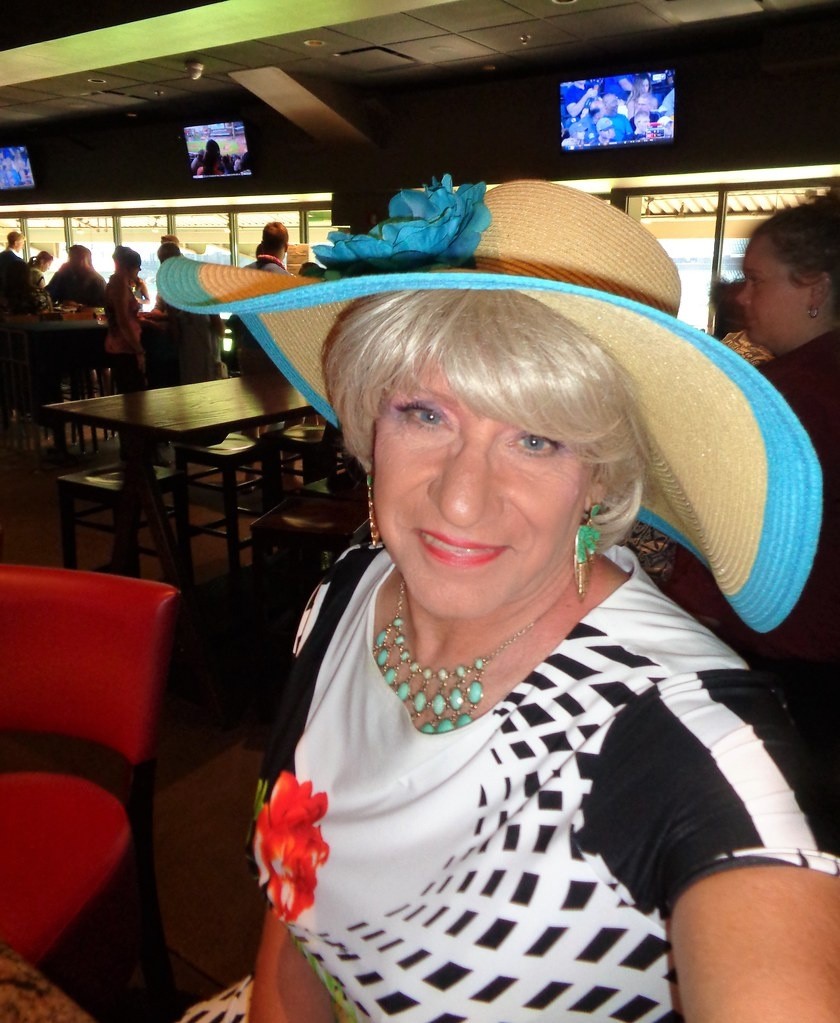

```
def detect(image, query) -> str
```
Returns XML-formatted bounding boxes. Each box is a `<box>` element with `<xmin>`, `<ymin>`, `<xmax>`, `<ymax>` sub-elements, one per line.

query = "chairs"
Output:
<box><xmin>0</xmin><ymin>562</ymin><xmax>182</xmax><ymax>980</ymax></box>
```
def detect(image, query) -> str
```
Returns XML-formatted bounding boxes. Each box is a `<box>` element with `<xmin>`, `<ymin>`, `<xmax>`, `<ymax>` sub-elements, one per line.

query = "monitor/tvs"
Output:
<box><xmin>183</xmin><ymin>117</ymin><xmax>255</xmax><ymax>181</ymax></box>
<box><xmin>557</xmin><ymin>67</ymin><xmax>681</xmax><ymax>155</ymax></box>
<box><xmin>0</xmin><ymin>145</ymin><xmax>37</xmax><ymax>192</ymax></box>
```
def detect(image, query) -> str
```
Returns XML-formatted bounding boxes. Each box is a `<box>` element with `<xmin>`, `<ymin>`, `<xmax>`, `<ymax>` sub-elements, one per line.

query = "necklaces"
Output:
<box><xmin>257</xmin><ymin>254</ymin><xmax>287</xmax><ymax>270</ymax></box>
<box><xmin>373</xmin><ymin>577</ymin><xmax>534</xmax><ymax>734</ymax></box>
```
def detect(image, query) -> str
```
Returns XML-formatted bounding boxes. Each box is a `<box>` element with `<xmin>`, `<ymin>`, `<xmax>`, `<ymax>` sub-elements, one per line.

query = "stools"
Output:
<box><xmin>174</xmin><ymin>434</ymin><xmax>266</xmax><ymax>576</ymax></box>
<box><xmin>282</xmin><ymin>419</ymin><xmax>343</xmax><ymax>553</ymax></box>
<box><xmin>57</xmin><ymin>466</ymin><xmax>187</xmax><ymax>576</ymax></box>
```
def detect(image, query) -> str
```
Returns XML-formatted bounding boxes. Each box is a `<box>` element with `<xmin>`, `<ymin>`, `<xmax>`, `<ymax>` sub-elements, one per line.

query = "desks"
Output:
<box><xmin>44</xmin><ymin>366</ymin><xmax>341</xmax><ymax>450</ymax></box>
<box><xmin>6</xmin><ymin>316</ymin><xmax>160</xmax><ymax>351</ymax></box>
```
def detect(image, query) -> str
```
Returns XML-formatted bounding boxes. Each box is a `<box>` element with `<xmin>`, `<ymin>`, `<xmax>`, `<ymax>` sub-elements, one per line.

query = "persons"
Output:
<box><xmin>102</xmin><ymin>246</ymin><xmax>147</xmax><ymax>395</ymax></box>
<box><xmin>29</xmin><ymin>251</ymin><xmax>54</xmax><ymax>287</ymax></box>
<box><xmin>669</xmin><ymin>185</ymin><xmax>840</xmax><ymax>663</ymax></box>
<box><xmin>242</xmin><ymin>222</ymin><xmax>290</xmax><ymax>274</ymax></box>
<box><xmin>0</xmin><ymin>259</ymin><xmax>52</xmax><ymax>315</ymax></box>
<box><xmin>561</xmin><ymin>72</ymin><xmax>674</xmax><ymax>146</ymax></box>
<box><xmin>149</xmin><ymin>233</ymin><xmax>225</xmax><ymax>385</ymax></box>
<box><xmin>155</xmin><ymin>180</ymin><xmax>840</xmax><ymax>1023</ymax></box>
<box><xmin>132</xmin><ymin>277</ymin><xmax>151</xmax><ymax>311</ymax></box>
<box><xmin>191</xmin><ymin>139</ymin><xmax>249</xmax><ymax>175</ymax></box>
<box><xmin>0</xmin><ymin>231</ymin><xmax>21</xmax><ymax>262</ymax></box>
<box><xmin>46</xmin><ymin>244</ymin><xmax>106</xmax><ymax>307</ymax></box>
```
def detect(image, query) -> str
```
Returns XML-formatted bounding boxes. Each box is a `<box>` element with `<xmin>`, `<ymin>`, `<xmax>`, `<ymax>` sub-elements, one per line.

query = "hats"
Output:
<box><xmin>112</xmin><ymin>245</ymin><xmax>142</xmax><ymax>269</ymax></box>
<box><xmin>155</xmin><ymin>177</ymin><xmax>825</xmax><ymax>636</ymax></box>
